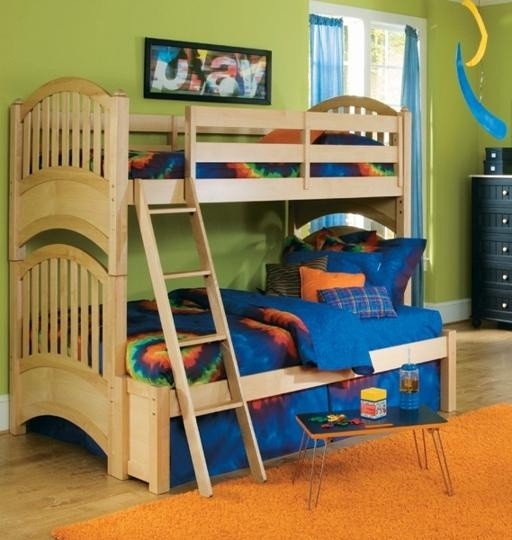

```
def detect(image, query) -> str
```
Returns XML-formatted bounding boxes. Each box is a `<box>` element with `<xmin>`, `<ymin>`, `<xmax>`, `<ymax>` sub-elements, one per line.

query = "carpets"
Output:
<box><xmin>43</xmin><ymin>401</ymin><xmax>512</xmax><ymax>540</ymax></box>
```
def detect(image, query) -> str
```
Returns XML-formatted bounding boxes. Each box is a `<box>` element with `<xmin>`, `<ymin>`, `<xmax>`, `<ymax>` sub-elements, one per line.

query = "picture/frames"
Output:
<box><xmin>140</xmin><ymin>36</ymin><xmax>271</xmax><ymax>106</ymax></box>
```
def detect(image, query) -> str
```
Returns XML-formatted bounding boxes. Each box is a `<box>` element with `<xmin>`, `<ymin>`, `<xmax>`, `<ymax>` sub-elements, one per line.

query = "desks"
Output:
<box><xmin>291</xmin><ymin>404</ymin><xmax>455</xmax><ymax>511</ymax></box>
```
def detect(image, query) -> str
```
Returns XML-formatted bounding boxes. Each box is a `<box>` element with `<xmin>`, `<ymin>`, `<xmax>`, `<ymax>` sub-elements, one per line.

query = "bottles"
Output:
<box><xmin>399</xmin><ymin>363</ymin><xmax>420</xmax><ymax>410</ymax></box>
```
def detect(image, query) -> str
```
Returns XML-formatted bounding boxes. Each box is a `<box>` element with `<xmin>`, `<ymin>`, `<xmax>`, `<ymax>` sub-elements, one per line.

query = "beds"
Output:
<box><xmin>8</xmin><ymin>76</ymin><xmax>458</xmax><ymax>494</ymax></box>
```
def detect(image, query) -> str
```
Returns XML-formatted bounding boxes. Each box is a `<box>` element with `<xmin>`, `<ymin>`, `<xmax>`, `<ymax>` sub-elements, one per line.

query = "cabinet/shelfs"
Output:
<box><xmin>470</xmin><ymin>178</ymin><xmax>512</xmax><ymax>330</ymax></box>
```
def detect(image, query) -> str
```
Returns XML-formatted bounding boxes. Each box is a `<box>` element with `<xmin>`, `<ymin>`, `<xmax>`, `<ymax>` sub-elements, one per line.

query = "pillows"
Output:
<box><xmin>259</xmin><ymin>238</ymin><xmax>428</xmax><ymax>320</ymax></box>
